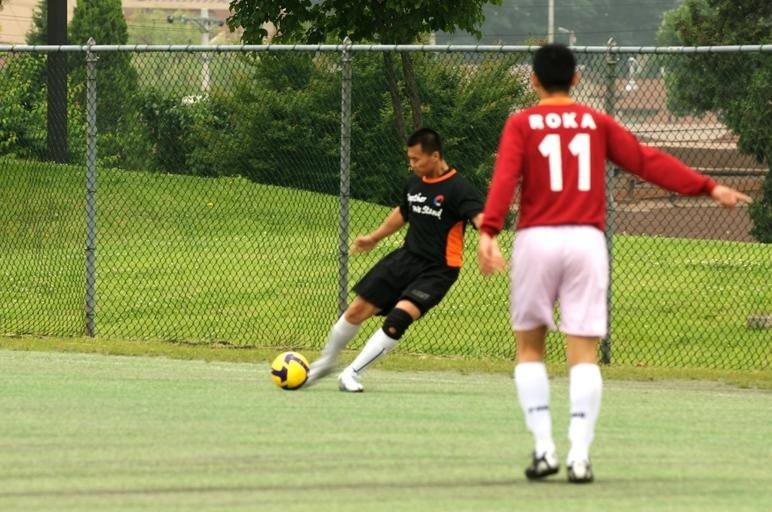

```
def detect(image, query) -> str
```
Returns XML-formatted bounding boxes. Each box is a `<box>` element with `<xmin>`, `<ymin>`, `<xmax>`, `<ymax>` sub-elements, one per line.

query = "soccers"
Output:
<box><xmin>270</xmin><ymin>352</ymin><xmax>309</xmax><ymax>391</ymax></box>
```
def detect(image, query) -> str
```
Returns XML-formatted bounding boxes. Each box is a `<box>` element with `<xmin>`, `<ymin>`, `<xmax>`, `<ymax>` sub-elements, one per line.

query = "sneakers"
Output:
<box><xmin>566</xmin><ymin>459</ymin><xmax>593</xmax><ymax>483</ymax></box>
<box><xmin>525</xmin><ymin>448</ymin><xmax>560</xmax><ymax>480</ymax></box>
<box><xmin>301</xmin><ymin>360</ymin><xmax>333</xmax><ymax>388</ymax></box>
<box><xmin>336</xmin><ymin>373</ymin><xmax>364</xmax><ymax>393</ymax></box>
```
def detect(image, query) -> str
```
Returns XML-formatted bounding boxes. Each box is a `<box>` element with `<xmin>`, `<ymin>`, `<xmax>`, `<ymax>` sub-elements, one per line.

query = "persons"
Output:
<box><xmin>304</xmin><ymin>126</ymin><xmax>508</xmax><ymax>393</ymax></box>
<box><xmin>476</xmin><ymin>39</ymin><xmax>756</xmax><ymax>485</ymax></box>
